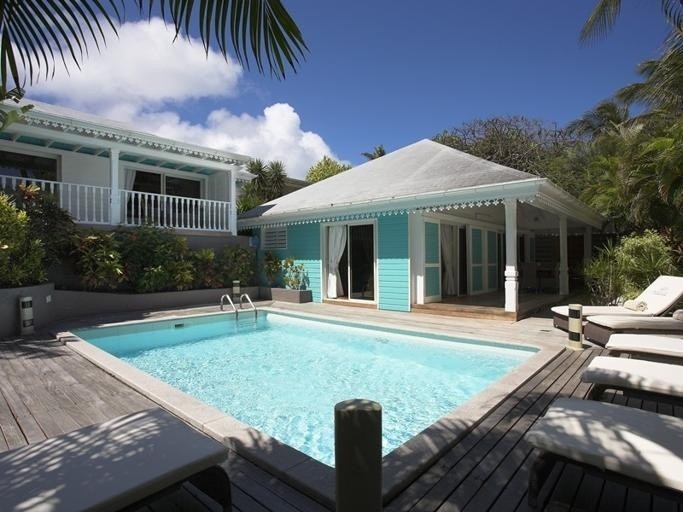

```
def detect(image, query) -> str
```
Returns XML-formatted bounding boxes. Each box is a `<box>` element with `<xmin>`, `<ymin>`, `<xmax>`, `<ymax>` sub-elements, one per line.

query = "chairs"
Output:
<box><xmin>524</xmin><ymin>397</ymin><xmax>683</xmax><ymax>507</ymax></box>
<box><xmin>580</xmin><ymin>356</ymin><xmax>682</xmax><ymax>406</ymax></box>
<box><xmin>585</xmin><ymin>306</ymin><xmax>683</xmax><ymax>347</ymax></box>
<box><xmin>551</xmin><ymin>275</ymin><xmax>683</xmax><ymax>334</ymax></box>
<box><xmin>605</xmin><ymin>334</ymin><xmax>683</xmax><ymax>357</ymax></box>
<box><xmin>0</xmin><ymin>407</ymin><xmax>230</xmax><ymax>512</ymax></box>
